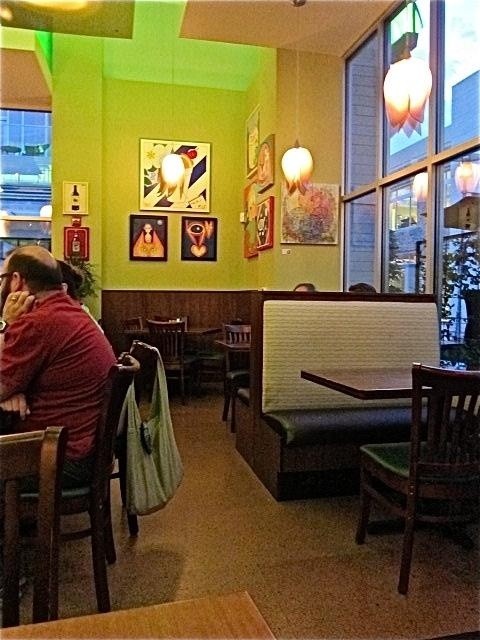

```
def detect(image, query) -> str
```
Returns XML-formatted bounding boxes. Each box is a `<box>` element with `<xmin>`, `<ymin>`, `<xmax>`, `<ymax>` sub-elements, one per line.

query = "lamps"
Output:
<box><xmin>157</xmin><ymin>0</ymin><xmax>194</xmax><ymax>199</ymax></box>
<box><xmin>282</xmin><ymin>0</ymin><xmax>314</xmax><ymax>196</ymax></box>
<box><xmin>39</xmin><ymin>206</ymin><xmax>51</xmax><ymax>235</ymax></box>
<box><xmin>384</xmin><ymin>0</ymin><xmax>432</xmax><ymax>133</ymax></box>
<box><xmin>455</xmin><ymin>158</ymin><xmax>479</xmax><ymax>198</ymax></box>
<box><xmin>413</xmin><ymin>173</ymin><xmax>428</xmax><ymax>206</ymax></box>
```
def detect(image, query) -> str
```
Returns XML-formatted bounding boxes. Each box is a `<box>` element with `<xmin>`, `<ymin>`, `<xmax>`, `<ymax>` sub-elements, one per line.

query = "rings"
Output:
<box><xmin>10</xmin><ymin>296</ymin><xmax>17</xmax><ymax>301</ymax></box>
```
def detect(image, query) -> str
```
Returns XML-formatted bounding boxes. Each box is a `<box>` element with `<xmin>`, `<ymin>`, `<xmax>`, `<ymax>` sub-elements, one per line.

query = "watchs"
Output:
<box><xmin>0</xmin><ymin>319</ymin><xmax>7</xmax><ymax>333</ymax></box>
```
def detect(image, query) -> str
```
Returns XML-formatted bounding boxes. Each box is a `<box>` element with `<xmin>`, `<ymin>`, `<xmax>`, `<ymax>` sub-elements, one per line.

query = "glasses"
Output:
<box><xmin>0</xmin><ymin>270</ymin><xmax>16</xmax><ymax>284</ymax></box>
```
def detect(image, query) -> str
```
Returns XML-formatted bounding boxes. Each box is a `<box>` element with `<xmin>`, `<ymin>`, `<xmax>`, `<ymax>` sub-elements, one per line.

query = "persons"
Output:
<box><xmin>293</xmin><ymin>283</ymin><xmax>314</xmax><ymax>294</ymax></box>
<box><xmin>349</xmin><ymin>283</ymin><xmax>375</xmax><ymax>292</ymax></box>
<box><xmin>0</xmin><ymin>245</ymin><xmax>118</xmax><ymax>492</ymax></box>
<box><xmin>54</xmin><ymin>259</ymin><xmax>104</xmax><ymax>334</ymax></box>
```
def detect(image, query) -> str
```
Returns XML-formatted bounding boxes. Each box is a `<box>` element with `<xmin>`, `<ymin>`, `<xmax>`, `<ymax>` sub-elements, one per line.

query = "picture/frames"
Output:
<box><xmin>245</xmin><ymin>103</ymin><xmax>259</xmax><ymax>178</ymax></box>
<box><xmin>254</xmin><ymin>133</ymin><xmax>274</xmax><ymax>193</ymax></box>
<box><xmin>64</xmin><ymin>216</ymin><xmax>89</xmax><ymax>260</ymax></box>
<box><xmin>62</xmin><ymin>181</ymin><xmax>89</xmax><ymax>214</ymax></box>
<box><xmin>181</xmin><ymin>215</ymin><xmax>218</xmax><ymax>262</ymax></box>
<box><xmin>244</xmin><ymin>174</ymin><xmax>257</xmax><ymax>258</ymax></box>
<box><xmin>138</xmin><ymin>138</ymin><xmax>212</xmax><ymax>213</ymax></box>
<box><xmin>258</xmin><ymin>195</ymin><xmax>274</xmax><ymax>250</ymax></box>
<box><xmin>281</xmin><ymin>183</ymin><xmax>340</xmax><ymax>245</ymax></box>
<box><xmin>130</xmin><ymin>214</ymin><xmax>168</xmax><ymax>262</ymax></box>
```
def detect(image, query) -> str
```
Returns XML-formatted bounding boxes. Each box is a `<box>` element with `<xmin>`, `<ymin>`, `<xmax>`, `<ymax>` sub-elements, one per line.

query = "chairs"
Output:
<box><xmin>147</xmin><ymin>320</ymin><xmax>197</xmax><ymax>405</ymax></box>
<box><xmin>224</xmin><ymin>324</ymin><xmax>252</xmax><ymax>373</ymax></box>
<box><xmin>355</xmin><ymin>362</ymin><xmax>480</xmax><ymax>594</ymax></box>
<box><xmin>196</xmin><ymin>317</ymin><xmax>243</xmax><ymax>392</ymax></box>
<box><xmin>221</xmin><ymin>371</ymin><xmax>251</xmax><ymax>431</ymax></box>
<box><xmin>121</xmin><ymin>316</ymin><xmax>143</xmax><ymax>330</ymax></box>
<box><xmin>98</xmin><ymin>341</ymin><xmax>159</xmax><ymax>537</ymax></box>
<box><xmin>155</xmin><ymin>315</ymin><xmax>188</xmax><ymax>332</ymax></box>
<box><xmin>0</xmin><ymin>426</ymin><xmax>91</xmax><ymax>627</ymax></box>
<box><xmin>18</xmin><ymin>352</ymin><xmax>140</xmax><ymax>612</ymax></box>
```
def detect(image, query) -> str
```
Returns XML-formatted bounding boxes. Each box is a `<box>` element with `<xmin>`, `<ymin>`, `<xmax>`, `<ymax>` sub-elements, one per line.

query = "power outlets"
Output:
<box><xmin>240</xmin><ymin>212</ymin><xmax>245</xmax><ymax>223</ymax></box>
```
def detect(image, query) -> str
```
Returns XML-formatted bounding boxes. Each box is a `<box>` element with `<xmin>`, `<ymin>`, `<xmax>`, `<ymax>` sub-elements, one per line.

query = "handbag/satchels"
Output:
<box><xmin>125</xmin><ymin>344</ymin><xmax>184</xmax><ymax>516</ymax></box>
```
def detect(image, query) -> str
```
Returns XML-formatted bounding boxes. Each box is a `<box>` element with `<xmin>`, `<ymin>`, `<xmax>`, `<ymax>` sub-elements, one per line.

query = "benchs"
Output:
<box><xmin>235</xmin><ymin>292</ymin><xmax>480</xmax><ymax>502</ymax></box>
